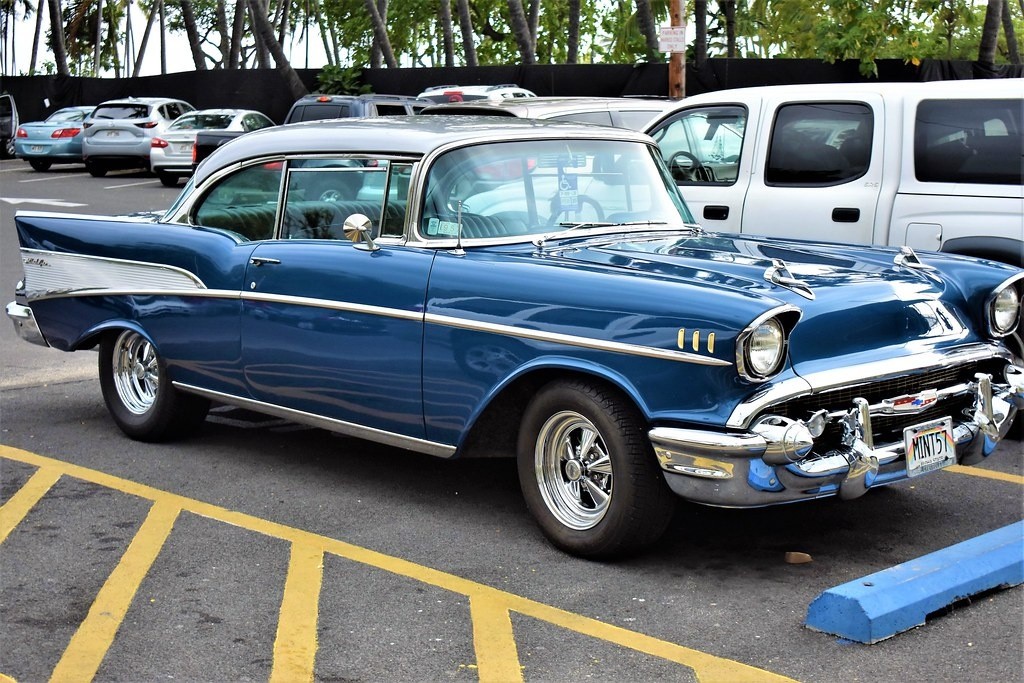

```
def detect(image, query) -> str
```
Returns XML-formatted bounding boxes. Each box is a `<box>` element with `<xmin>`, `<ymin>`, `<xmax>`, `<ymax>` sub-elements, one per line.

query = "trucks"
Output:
<box><xmin>192</xmin><ymin>83</ymin><xmax>533</xmax><ymax>209</ymax></box>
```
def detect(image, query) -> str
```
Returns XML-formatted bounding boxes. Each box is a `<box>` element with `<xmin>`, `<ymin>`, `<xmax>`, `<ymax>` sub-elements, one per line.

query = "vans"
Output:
<box><xmin>570</xmin><ymin>77</ymin><xmax>1024</xmax><ymax>282</ymax></box>
<box><xmin>0</xmin><ymin>94</ymin><xmax>21</xmax><ymax>160</ymax></box>
<box><xmin>414</xmin><ymin>96</ymin><xmax>746</xmax><ymax>236</ymax></box>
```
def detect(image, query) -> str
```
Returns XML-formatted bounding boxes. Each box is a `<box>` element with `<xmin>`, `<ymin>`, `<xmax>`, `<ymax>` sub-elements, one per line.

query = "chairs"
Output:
<box><xmin>421</xmin><ymin>210</ymin><xmax>557</xmax><ymax>241</ymax></box>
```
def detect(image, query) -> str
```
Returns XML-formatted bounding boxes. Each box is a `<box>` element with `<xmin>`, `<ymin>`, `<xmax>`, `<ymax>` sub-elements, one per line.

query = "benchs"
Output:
<box><xmin>201</xmin><ymin>200</ymin><xmax>424</xmax><ymax>242</ymax></box>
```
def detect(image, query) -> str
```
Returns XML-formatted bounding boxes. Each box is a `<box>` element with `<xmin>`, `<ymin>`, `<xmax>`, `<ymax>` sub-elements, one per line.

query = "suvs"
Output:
<box><xmin>82</xmin><ymin>96</ymin><xmax>197</xmax><ymax>179</ymax></box>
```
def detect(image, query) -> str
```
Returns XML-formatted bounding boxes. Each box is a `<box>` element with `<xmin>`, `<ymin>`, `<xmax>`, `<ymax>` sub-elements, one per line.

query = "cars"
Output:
<box><xmin>150</xmin><ymin>108</ymin><xmax>278</xmax><ymax>187</ymax></box>
<box><xmin>8</xmin><ymin>117</ymin><xmax>1024</xmax><ymax>566</ymax></box>
<box><xmin>14</xmin><ymin>105</ymin><xmax>100</xmax><ymax>171</ymax></box>
